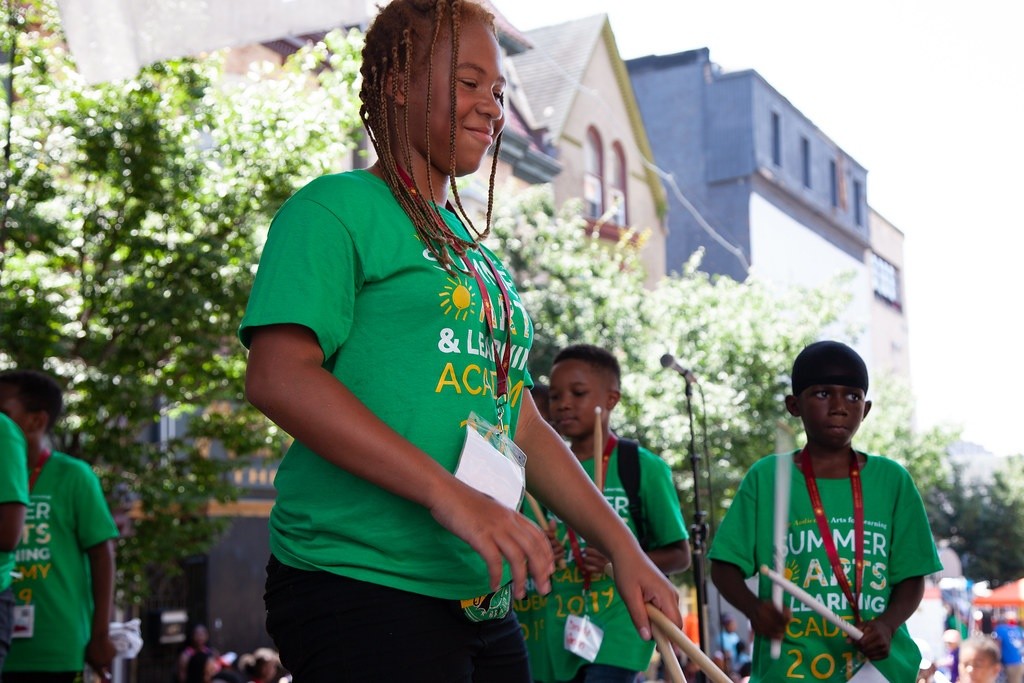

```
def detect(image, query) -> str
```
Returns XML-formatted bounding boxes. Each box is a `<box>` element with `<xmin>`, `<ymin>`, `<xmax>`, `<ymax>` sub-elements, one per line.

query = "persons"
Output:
<box><xmin>0</xmin><ymin>368</ymin><xmax>119</xmax><ymax>683</ymax></box>
<box><xmin>919</xmin><ymin>609</ymin><xmax>1024</xmax><ymax>683</ymax></box>
<box><xmin>704</xmin><ymin>339</ymin><xmax>944</xmax><ymax>683</ymax></box>
<box><xmin>178</xmin><ymin>624</ymin><xmax>289</xmax><ymax>683</ymax></box>
<box><xmin>511</xmin><ymin>344</ymin><xmax>691</xmax><ymax>683</ymax></box>
<box><xmin>237</xmin><ymin>0</ymin><xmax>685</xmax><ymax>683</ymax></box>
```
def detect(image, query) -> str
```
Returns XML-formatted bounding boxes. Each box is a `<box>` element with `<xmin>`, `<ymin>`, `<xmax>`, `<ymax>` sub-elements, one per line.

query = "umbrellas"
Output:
<box><xmin>972</xmin><ymin>578</ymin><xmax>1024</xmax><ymax>606</ymax></box>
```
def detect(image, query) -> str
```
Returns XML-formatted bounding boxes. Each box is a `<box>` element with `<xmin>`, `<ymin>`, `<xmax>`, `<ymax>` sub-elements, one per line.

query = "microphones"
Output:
<box><xmin>660</xmin><ymin>354</ymin><xmax>697</xmax><ymax>382</ymax></box>
